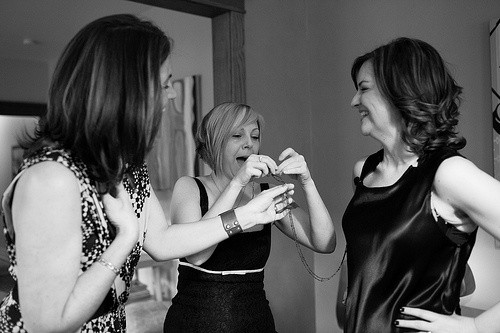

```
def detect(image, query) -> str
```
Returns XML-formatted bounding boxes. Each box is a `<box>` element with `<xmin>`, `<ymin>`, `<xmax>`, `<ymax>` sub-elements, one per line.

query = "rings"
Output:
<box><xmin>259</xmin><ymin>156</ymin><xmax>263</xmax><ymax>162</ymax></box>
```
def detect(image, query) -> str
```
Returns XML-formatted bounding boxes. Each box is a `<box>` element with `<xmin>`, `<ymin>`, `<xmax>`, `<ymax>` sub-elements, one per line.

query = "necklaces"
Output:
<box><xmin>209</xmin><ymin>174</ymin><xmax>245</xmax><ymax>208</ymax></box>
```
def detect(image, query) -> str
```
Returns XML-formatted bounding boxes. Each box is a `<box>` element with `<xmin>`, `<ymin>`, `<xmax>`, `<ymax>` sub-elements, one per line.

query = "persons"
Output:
<box><xmin>163</xmin><ymin>101</ymin><xmax>336</xmax><ymax>333</ymax></box>
<box><xmin>341</xmin><ymin>36</ymin><xmax>500</xmax><ymax>333</ymax></box>
<box><xmin>0</xmin><ymin>13</ymin><xmax>295</xmax><ymax>333</ymax></box>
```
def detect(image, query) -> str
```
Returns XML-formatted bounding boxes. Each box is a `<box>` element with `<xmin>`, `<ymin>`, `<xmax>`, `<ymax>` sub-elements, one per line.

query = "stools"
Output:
<box><xmin>135</xmin><ymin>251</ymin><xmax>174</xmax><ymax>303</ymax></box>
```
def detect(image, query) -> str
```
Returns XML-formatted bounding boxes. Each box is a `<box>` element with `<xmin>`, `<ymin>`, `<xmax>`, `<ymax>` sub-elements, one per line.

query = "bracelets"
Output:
<box><xmin>218</xmin><ymin>208</ymin><xmax>243</xmax><ymax>238</ymax></box>
<box><xmin>97</xmin><ymin>257</ymin><xmax>120</xmax><ymax>275</ymax></box>
